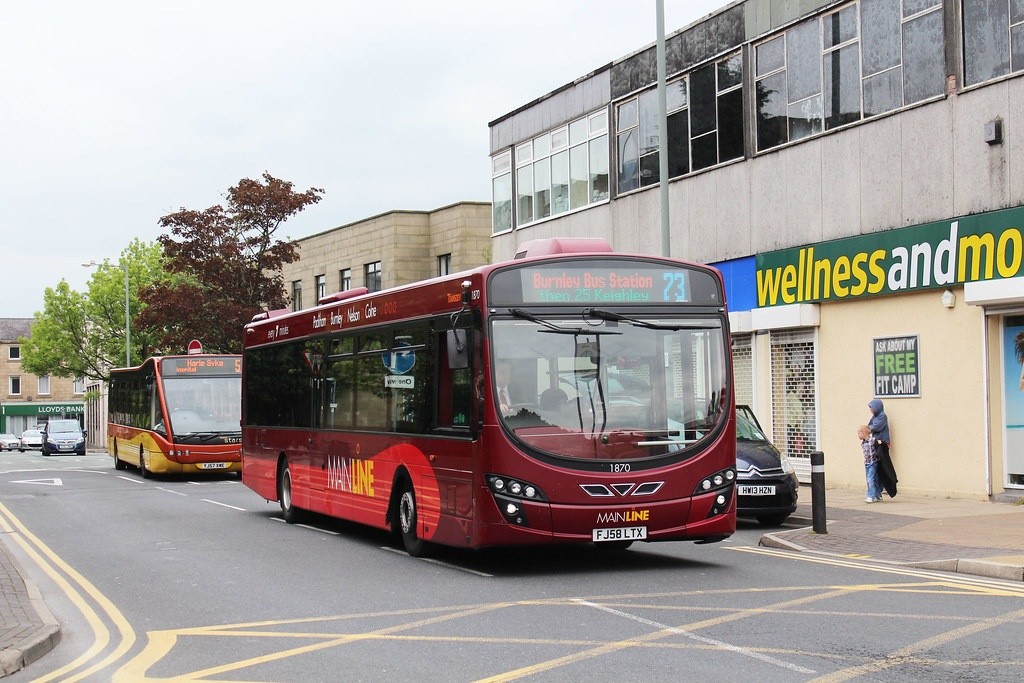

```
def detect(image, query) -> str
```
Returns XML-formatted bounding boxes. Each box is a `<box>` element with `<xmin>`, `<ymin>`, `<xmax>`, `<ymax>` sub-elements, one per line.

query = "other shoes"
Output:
<box><xmin>875</xmin><ymin>499</ymin><xmax>884</xmax><ymax>502</ymax></box>
<box><xmin>865</xmin><ymin>498</ymin><xmax>875</xmax><ymax>503</ymax></box>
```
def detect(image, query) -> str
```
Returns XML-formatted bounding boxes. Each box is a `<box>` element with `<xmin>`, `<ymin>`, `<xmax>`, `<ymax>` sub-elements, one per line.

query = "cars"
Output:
<box><xmin>592</xmin><ymin>373</ymin><xmax>800</xmax><ymax>531</ymax></box>
<box><xmin>18</xmin><ymin>429</ymin><xmax>46</xmax><ymax>452</ymax></box>
<box><xmin>0</xmin><ymin>433</ymin><xmax>22</xmax><ymax>452</ymax></box>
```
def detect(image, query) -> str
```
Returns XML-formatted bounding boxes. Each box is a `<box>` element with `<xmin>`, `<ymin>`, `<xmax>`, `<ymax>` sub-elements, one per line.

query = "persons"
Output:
<box><xmin>496</xmin><ymin>362</ymin><xmax>524</xmax><ymax>414</ymax></box>
<box><xmin>858</xmin><ymin>425</ymin><xmax>883</xmax><ymax>502</ymax></box>
<box><xmin>868</xmin><ymin>399</ymin><xmax>892</xmax><ymax>449</ymax></box>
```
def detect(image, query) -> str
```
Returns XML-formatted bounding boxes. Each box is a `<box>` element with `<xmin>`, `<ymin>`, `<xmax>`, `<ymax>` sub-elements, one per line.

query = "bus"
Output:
<box><xmin>240</xmin><ymin>234</ymin><xmax>738</xmax><ymax>559</ymax></box>
<box><xmin>107</xmin><ymin>354</ymin><xmax>242</xmax><ymax>480</ymax></box>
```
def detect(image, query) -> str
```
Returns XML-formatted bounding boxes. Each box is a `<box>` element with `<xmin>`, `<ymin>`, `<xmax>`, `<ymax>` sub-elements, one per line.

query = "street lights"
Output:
<box><xmin>81</xmin><ymin>262</ymin><xmax>130</xmax><ymax>367</ymax></box>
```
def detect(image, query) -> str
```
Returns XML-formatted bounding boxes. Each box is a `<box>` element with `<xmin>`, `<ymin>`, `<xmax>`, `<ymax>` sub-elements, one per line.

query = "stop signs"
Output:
<box><xmin>187</xmin><ymin>339</ymin><xmax>203</xmax><ymax>356</ymax></box>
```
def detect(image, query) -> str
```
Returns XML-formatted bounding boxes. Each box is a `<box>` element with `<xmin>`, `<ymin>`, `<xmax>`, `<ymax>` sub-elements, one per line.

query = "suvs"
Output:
<box><xmin>40</xmin><ymin>418</ymin><xmax>89</xmax><ymax>456</ymax></box>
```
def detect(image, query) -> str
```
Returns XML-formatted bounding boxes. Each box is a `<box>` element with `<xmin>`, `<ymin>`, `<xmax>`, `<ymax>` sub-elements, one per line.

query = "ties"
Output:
<box><xmin>499</xmin><ymin>390</ymin><xmax>508</xmax><ymax>406</ymax></box>
